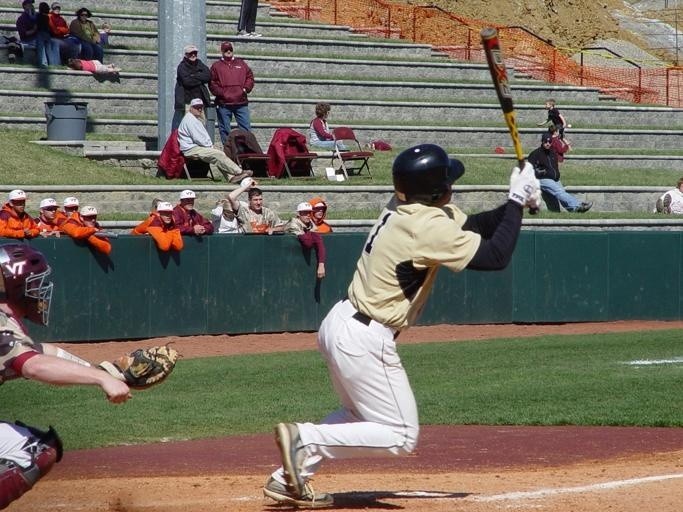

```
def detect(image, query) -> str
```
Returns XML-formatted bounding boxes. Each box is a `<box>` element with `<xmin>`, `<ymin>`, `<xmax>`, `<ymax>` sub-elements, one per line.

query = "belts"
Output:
<box><xmin>342</xmin><ymin>294</ymin><xmax>399</xmax><ymax>340</ymax></box>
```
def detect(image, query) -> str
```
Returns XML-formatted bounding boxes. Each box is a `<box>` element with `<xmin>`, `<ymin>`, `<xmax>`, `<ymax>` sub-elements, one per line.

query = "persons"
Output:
<box><xmin>208</xmin><ymin>41</ymin><xmax>254</xmax><ymax>151</ymax></box>
<box><xmin>177</xmin><ymin>96</ymin><xmax>254</xmax><ymax>184</ymax></box>
<box><xmin>285</xmin><ymin>197</ymin><xmax>331</xmax><ymax>278</ymax></box>
<box><xmin>536</xmin><ymin>101</ymin><xmax>565</xmax><ymax>136</ymax></box>
<box><xmin>261</xmin><ymin>144</ymin><xmax>538</xmax><ymax>509</ymax></box>
<box><xmin>210</xmin><ymin>185</ymin><xmax>286</xmax><ymax>235</ymax></box>
<box><xmin>0</xmin><ymin>189</ymin><xmax>112</xmax><ymax>254</ymax></box>
<box><xmin>656</xmin><ymin>177</ymin><xmax>682</xmax><ymax>213</ymax></box>
<box><xmin>0</xmin><ymin>242</ymin><xmax>137</xmax><ymax>509</ymax></box>
<box><xmin>14</xmin><ymin>2</ymin><xmax>119</xmax><ymax>80</ymax></box>
<box><xmin>172</xmin><ymin>46</ymin><xmax>216</xmax><ymax>151</ymax></box>
<box><xmin>547</xmin><ymin>126</ymin><xmax>569</xmax><ymax>163</ymax></box>
<box><xmin>529</xmin><ymin>132</ymin><xmax>592</xmax><ymax>213</ymax></box>
<box><xmin>131</xmin><ymin>188</ymin><xmax>213</xmax><ymax>249</ymax></box>
<box><xmin>307</xmin><ymin>98</ymin><xmax>350</xmax><ymax>154</ymax></box>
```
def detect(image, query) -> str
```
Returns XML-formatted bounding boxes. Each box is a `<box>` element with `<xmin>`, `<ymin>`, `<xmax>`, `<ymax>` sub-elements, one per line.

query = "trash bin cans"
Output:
<box><xmin>43</xmin><ymin>101</ymin><xmax>88</xmax><ymax>140</ymax></box>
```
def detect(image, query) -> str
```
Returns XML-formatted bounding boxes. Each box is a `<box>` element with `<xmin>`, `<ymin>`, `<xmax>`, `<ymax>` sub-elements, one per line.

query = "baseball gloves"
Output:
<box><xmin>98</xmin><ymin>345</ymin><xmax>180</xmax><ymax>389</ymax></box>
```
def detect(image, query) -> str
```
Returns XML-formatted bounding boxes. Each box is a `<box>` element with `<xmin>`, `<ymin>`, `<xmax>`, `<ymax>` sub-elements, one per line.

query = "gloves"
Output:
<box><xmin>507</xmin><ymin>162</ymin><xmax>541</xmax><ymax>208</ymax></box>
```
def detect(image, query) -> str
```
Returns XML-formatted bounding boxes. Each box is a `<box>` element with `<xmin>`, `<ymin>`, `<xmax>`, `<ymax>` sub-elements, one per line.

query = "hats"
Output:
<box><xmin>156</xmin><ymin>202</ymin><xmax>175</xmax><ymax>212</ymax></box>
<box><xmin>221</xmin><ymin>42</ymin><xmax>233</xmax><ymax>50</ymax></box>
<box><xmin>79</xmin><ymin>205</ymin><xmax>98</xmax><ymax>217</ymax></box>
<box><xmin>190</xmin><ymin>98</ymin><xmax>205</xmax><ymax>108</ymax></box>
<box><xmin>179</xmin><ymin>190</ymin><xmax>196</xmax><ymax>200</ymax></box>
<box><xmin>297</xmin><ymin>202</ymin><xmax>326</xmax><ymax>212</ymax></box>
<box><xmin>8</xmin><ymin>190</ymin><xmax>30</xmax><ymax>202</ymax></box>
<box><xmin>63</xmin><ymin>197</ymin><xmax>79</xmax><ymax>209</ymax></box>
<box><xmin>39</xmin><ymin>197</ymin><xmax>60</xmax><ymax>211</ymax></box>
<box><xmin>184</xmin><ymin>45</ymin><xmax>198</xmax><ymax>54</ymax></box>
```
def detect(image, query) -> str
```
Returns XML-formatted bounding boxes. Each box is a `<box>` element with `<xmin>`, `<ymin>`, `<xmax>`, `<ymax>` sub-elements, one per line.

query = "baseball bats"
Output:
<box><xmin>481</xmin><ymin>29</ymin><xmax>541</xmax><ymax>216</ymax></box>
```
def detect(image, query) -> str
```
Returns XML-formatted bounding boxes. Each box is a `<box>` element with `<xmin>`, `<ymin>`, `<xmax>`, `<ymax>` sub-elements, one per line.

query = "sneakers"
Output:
<box><xmin>275</xmin><ymin>422</ymin><xmax>304</xmax><ymax>497</ymax></box>
<box><xmin>576</xmin><ymin>202</ymin><xmax>592</xmax><ymax>212</ymax></box>
<box><xmin>229</xmin><ymin>169</ymin><xmax>253</xmax><ymax>183</ymax></box>
<box><xmin>262</xmin><ymin>476</ymin><xmax>333</xmax><ymax>509</ymax></box>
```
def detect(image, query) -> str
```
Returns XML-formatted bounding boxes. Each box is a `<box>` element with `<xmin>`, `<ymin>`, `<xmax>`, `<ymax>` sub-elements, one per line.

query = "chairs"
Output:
<box><xmin>227</xmin><ymin>129</ymin><xmax>272</xmax><ymax>182</ymax></box>
<box><xmin>268</xmin><ymin>127</ymin><xmax>316</xmax><ymax>180</ymax></box>
<box><xmin>171</xmin><ymin>128</ymin><xmax>216</xmax><ymax>181</ymax></box>
<box><xmin>328</xmin><ymin>127</ymin><xmax>375</xmax><ymax>182</ymax></box>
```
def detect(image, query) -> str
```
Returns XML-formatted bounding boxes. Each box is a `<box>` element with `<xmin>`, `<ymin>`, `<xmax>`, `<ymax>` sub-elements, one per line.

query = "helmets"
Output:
<box><xmin>0</xmin><ymin>244</ymin><xmax>53</xmax><ymax>328</ymax></box>
<box><xmin>391</xmin><ymin>144</ymin><xmax>464</xmax><ymax>206</ymax></box>
